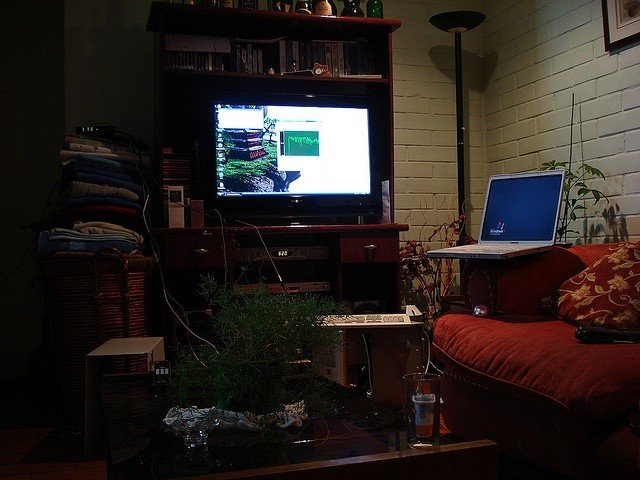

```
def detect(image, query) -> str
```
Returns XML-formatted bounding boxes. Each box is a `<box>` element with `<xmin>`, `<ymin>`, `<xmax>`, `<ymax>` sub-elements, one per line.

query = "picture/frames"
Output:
<box><xmin>601</xmin><ymin>0</ymin><xmax>640</xmax><ymax>52</ymax></box>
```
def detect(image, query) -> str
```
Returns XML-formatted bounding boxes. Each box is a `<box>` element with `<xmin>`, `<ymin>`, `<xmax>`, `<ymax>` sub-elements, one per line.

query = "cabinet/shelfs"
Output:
<box><xmin>86</xmin><ymin>337</ymin><xmax>165</xmax><ymax>461</ymax></box>
<box><xmin>146</xmin><ymin>1</ymin><xmax>401</xmax><ymax>344</ymax></box>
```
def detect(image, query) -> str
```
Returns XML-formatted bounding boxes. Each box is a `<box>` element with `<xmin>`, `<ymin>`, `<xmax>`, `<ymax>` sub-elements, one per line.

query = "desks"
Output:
<box><xmin>307</xmin><ymin>313</ymin><xmax>424</xmax><ymax>419</ymax></box>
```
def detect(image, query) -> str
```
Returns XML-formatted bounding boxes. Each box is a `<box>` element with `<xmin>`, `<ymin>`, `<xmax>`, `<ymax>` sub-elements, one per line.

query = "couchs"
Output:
<box><xmin>433</xmin><ymin>243</ymin><xmax>640</xmax><ymax>479</ymax></box>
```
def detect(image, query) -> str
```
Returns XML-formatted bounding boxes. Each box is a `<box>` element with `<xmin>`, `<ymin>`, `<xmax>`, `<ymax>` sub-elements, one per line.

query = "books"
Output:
<box><xmin>158</xmin><ymin>153</ymin><xmax>195</xmax><ymax>226</ymax></box>
<box><xmin>160</xmin><ymin>30</ymin><xmax>388</xmax><ymax>78</ymax></box>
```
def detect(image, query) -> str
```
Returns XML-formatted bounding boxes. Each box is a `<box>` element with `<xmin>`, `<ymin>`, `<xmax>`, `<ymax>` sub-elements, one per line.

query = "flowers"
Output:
<box><xmin>399</xmin><ymin>205</ymin><xmax>476</xmax><ymax>315</ymax></box>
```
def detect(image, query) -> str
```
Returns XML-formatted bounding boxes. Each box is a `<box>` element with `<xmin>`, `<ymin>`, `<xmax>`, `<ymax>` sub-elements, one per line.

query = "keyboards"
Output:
<box><xmin>297</xmin><ymin>313</ymin><xmax>412</xmax><ymax>327</ymax></box>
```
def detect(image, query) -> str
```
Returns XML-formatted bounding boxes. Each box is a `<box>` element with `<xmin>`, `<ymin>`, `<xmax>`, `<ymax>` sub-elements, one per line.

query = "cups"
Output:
<box><xmin>403</xmin><ymin>374</ymin><xmax>441</xmax><ymax>449</ymax></box>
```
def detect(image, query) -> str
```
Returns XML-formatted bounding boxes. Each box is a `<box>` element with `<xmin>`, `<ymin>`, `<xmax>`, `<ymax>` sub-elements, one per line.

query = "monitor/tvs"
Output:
<box><xmin>198</xmin><ymin>91</ymin><xmax>380</xmax><ymax>226</ymax></box>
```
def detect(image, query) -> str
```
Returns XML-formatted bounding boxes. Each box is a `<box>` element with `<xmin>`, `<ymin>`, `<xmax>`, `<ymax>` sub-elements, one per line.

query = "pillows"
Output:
<box><xmin>557</xmin><ymin>241</ymin><xmax>640</xmax><ymax>331</ymax></box>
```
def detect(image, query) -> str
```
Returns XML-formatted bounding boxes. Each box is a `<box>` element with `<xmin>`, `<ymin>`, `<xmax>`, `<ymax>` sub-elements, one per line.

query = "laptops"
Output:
<box><xmin>425</xmin><ymin>169</ymin><xmax>566</xmax><ymax>259</ymax></box>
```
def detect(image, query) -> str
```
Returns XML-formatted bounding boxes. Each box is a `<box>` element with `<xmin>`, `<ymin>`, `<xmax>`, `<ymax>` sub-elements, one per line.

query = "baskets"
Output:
<box><xmin>35</xmin><ymin>246</ymin><xmax>157</xmax><ymax>430</ymax></box>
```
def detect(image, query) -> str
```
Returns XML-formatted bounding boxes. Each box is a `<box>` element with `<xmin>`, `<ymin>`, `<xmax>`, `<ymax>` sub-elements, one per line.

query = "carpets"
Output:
<box><xmin>18</xmin><ymin>427</ymin><xmax>107</xmax><ymax>463</ymax></box>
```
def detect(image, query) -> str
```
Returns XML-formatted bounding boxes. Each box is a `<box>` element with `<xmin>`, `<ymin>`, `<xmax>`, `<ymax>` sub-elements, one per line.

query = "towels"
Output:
<box><xmin>37</xmin><ymin>130</ymin><xmax>145</xmax><ymax>256</ymax></box>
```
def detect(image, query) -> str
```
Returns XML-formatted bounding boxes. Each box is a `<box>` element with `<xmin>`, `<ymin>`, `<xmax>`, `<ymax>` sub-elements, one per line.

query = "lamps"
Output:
<box><xmin>430</xmin><ymin>10</ymin><xmax>486</xmax><ymax>296</ymax></box>
